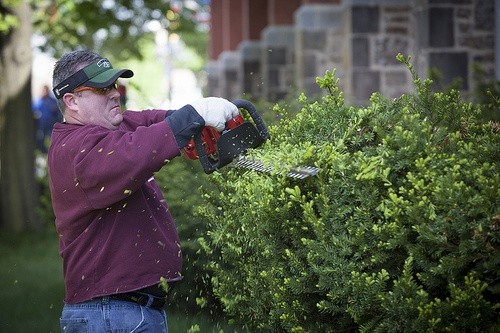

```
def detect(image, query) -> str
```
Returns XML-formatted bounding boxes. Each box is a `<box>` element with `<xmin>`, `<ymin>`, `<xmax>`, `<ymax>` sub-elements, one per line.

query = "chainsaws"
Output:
<box><xmin>179</xmin><ymin>97</ymin><xmax>322</xmax><ymax>184</ymax></box>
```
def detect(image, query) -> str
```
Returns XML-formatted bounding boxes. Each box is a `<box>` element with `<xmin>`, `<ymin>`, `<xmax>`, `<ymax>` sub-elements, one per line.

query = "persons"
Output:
<box><xmin>32</xmin><ymin>85</ymin><xmax>64</xmax><ymax>154</ymax></box>
<box><xmin>47</xmin><ymin>51</ymin><xmax>240</xmax><ymax>333</ymax></box>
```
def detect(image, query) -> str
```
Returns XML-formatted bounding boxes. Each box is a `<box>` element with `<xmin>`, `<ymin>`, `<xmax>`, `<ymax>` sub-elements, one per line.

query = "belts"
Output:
<box><xmin>106</xmin><ymin>292</ymin><xmax>169</xmax><ymax>310</ymax></box>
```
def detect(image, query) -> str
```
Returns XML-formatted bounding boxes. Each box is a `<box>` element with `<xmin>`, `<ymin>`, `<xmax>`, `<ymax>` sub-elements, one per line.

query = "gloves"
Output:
<box><xmin>189</xmin><ymin>97</ymin><xmax>240</xmax><ymax>133</ymax></box>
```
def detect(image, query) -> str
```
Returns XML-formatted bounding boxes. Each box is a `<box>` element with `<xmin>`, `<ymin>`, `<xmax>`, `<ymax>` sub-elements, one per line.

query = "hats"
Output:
<box><xmin>53</xmin><ymin>57</ymin><xmax>134</xmax><ymax>99</ymax></box>
